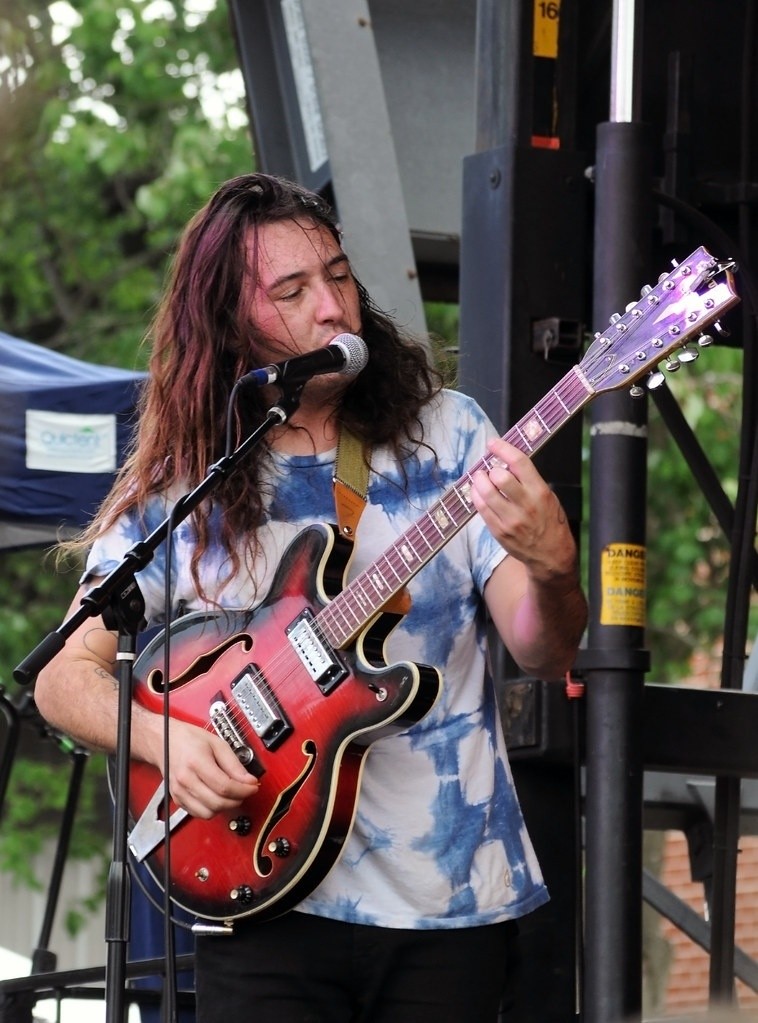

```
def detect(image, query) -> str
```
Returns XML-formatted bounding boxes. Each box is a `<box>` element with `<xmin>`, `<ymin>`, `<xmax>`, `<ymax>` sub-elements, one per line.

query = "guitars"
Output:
<box><xmin>106</xmin><ymin>244</ymin><xmax>740</xmax><ymax>929</ymax></box>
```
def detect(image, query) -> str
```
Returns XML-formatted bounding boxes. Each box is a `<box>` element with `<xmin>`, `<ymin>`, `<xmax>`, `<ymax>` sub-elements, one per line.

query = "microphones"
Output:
<box><xmin>237</xmin><ymin>332</ymin><xmax>369</xmax><ymax>388</ymax></box>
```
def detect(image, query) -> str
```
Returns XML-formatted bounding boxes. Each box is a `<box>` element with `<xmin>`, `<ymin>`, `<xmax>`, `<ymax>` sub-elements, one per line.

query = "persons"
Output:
<box><xmin>30</xmin><ymin>176</ymin><xmax>589</xmax><ymax>1022</ymax></box>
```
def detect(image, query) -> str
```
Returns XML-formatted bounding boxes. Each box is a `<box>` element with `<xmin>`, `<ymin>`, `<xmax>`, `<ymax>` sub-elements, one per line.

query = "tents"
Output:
<box><xmin>0</xmin><ymin>331</ymin><xmax>159</xmax><ymax>558</ymax></box>
